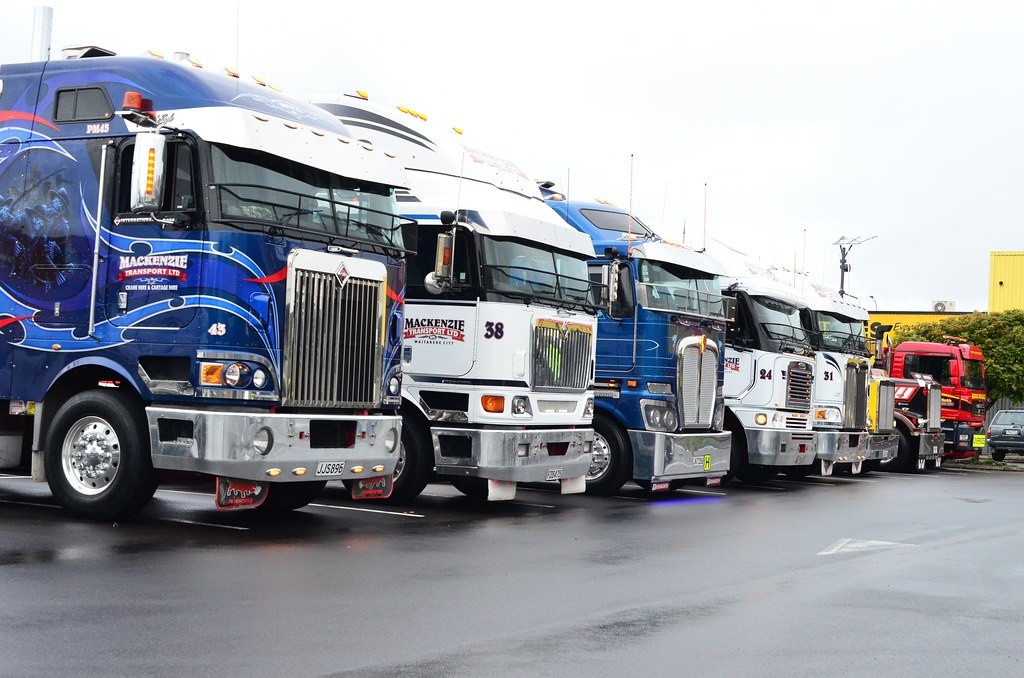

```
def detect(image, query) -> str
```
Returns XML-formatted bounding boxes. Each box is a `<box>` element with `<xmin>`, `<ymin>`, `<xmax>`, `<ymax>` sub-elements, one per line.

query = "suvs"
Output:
<box><xmin>987</xmin><ymin>407</ymin><xmax>1024</xmax><ymax>461</ymax></box>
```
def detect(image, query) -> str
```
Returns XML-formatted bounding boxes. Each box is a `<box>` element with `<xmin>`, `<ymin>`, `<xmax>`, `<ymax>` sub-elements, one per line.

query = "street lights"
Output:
<box><xmin>832</xmin><ymin>234</ymin><xmax>878</xmax><ymax>299</ymax></box>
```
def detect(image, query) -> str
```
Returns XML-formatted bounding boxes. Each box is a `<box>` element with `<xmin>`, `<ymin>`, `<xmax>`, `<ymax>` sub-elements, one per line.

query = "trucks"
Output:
<box><xmin>535</xmin><ymin>177</ymin><xmax>736</xmax><ymax>502</ymax></box>
<box><xmin>309</xmin><ymin>91</ymin><xmax>603</xmax><ymax>514</ymax></box>
<box><xmin>0</xmin><ymin>45</ymin><xmax>404</xmax><ymax>523</ymax></box>
<box><xmin>722</xmin><ymin>258</ymin><xmax>986</xmax><ymax>488</ymax></box>
